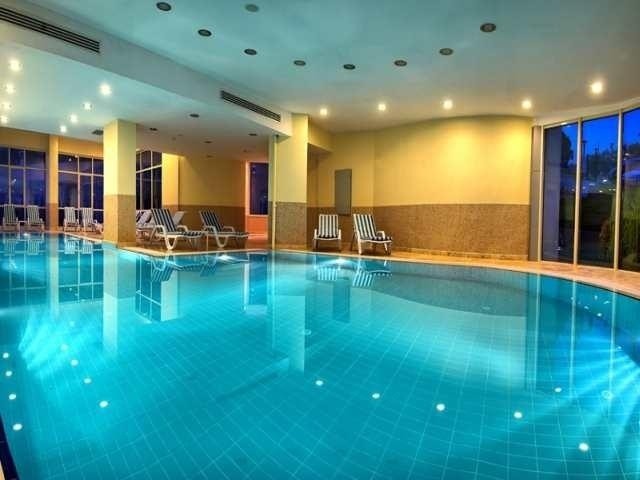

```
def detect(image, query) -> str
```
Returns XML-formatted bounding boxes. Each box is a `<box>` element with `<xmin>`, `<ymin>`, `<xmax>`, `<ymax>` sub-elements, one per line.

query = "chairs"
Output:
<box><xmin>352</xmin><ymin>257</ymin><xmax>392</xmax><ymax>289</ymax></box>
<box><xmin>82</xmin><ymin>207</ymin><xmax>98</xmax><ymax>232</ymax></box>
<box><xmin>63</xmin><ymin>207</ymin><xmax>80</xmax><ymax>231</ymax></box>
<box><xmin>350</xmin><ymin>213</ymin><xmax>393</xmax><ymax>256</ymax></box>
<box><xmin>2</xmin><ymin>203</ymin><xmax>20</xmax><ymax>231</ymax></box>
<box><xmin>3</xmin><ymin>232</ymin><xmax>93</xmax><ymax>257</ymax></box>
<box><xmin>313</xmin><ymin>255</ymin><xmax>341</xmax><ymax>281</ymax></box>
<box><xmin>200</xmin><ymin>253</ymin><xmax>250</xmax><ymax>278</ymax></box>
<box><xmin>151</xmin><ymin>256</ymin><xmax>204</xmax><ymax>282</ymax></box>
<box><xmin>26</xmin><ymin>205</ymin><xmax>44</xmax><ymax>231</ymax></box>
<box><xmin>99</xmin><ymin>208</ymin><xmax>249</xmax><ymax>251</ymax></box>
<box><xmin>313</xmin><ymin>214</ymin><xmax>342</xmax><ymax>251</ymax></box>
